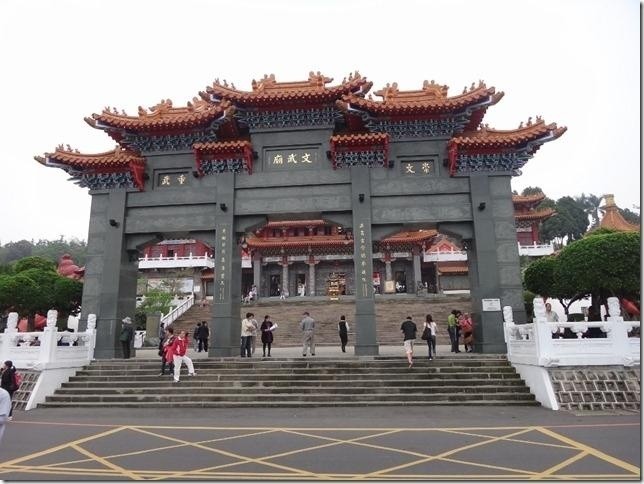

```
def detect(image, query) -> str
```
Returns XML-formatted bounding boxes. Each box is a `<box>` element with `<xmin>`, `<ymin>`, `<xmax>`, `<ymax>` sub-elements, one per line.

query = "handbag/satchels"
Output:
<box><xmin>15</xmin><ymin>371</ymin><xmax>22</xmax><ymax>387</ymax></box>
<box><xmin>421</xmin><ymin>328</ymin><xmax>431</xmax><ymax>340</ymax></box>
<box><xmin>119</xmin><ymin>331</ymin><xmax>129</xmax><ymax>342</ymax></box>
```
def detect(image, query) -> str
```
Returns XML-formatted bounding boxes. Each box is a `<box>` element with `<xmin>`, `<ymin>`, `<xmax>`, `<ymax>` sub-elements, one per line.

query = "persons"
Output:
<box><xmin>240</xmin><ymin>313</ymin><xmax>256</xmax><ymax>358</ymax></box>
<box><xmin>0</xmin><ymin>360</ymin><xmax>18</xmax><ymax>446</ymax></box>
<box><xmin>542</xmin><ymin>302</ymin><xmax>561</xmax><ymax>339</ymax></box>
<box><xmin>259</xmin><ymin>314</ymin><xmax>275</xmax><ymax>358</ymax></box>
<box><xmin>156</xmin><ymin>320</ymin><xmax>210</xmax><ymax>384</ymax></box>
<box><xmin>240</xmin><ymin>284</ymin><xmax>258</xmax><ymax>306</ymax></box>
<box><xmin>399</xmin><ymin>316</ymin><xmax>418</xmax><ymax>371</ymax></box>
<box><xmin>445</xmin><ymin>308</ymin><xmax>475</xmax><ymax>354</ymax></box>
<box><xmin>298</xmin><ymin>311</ymin><xmax>318</xmax><ymax>357</ymax></box>
<box><xmin>420</xmin><ymin>313</ymin><xmax>441</xmax><ymax>361</ymax></box>
<box><xmin>199</xmin><ymin>296</ymin><xmax>209</xmax><ymax>309</ymax></box>
<box><xmin>243</xmin><ymin>313</ymin><xmax>259</xmax><ymax>357</ymax></box>
<box><xmin>118</xmin><ymin>316</ymin><xmax>134</xmax><ymax>360</ymax></box>
<box><xmin>336</xmin><ymin>314</ymin><xmax>350</xmax><ymax>353</ymax></box>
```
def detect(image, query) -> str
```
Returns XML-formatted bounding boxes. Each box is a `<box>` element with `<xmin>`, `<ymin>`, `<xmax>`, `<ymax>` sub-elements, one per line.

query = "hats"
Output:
<box><xmin>122</xmin><ymin>317</ymin><xmax>132</xmax><ymax>324</ymax></box>
